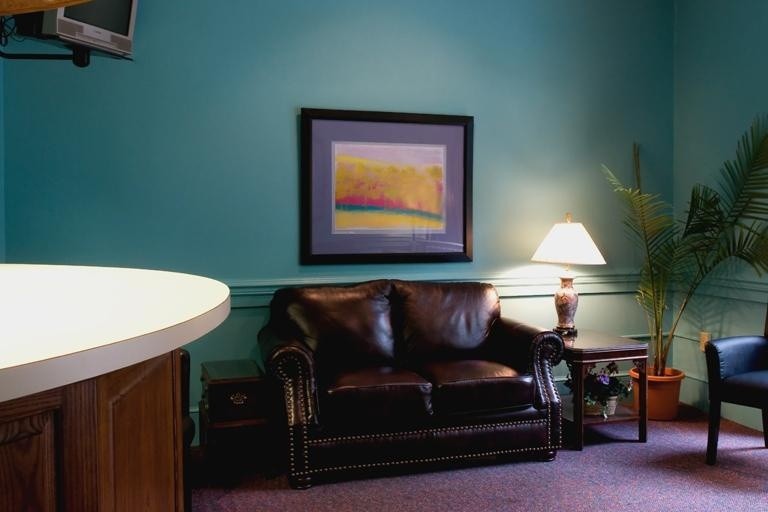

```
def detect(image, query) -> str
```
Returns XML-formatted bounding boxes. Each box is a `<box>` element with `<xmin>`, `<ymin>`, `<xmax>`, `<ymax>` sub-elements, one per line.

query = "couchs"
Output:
<box><xmin>256</xmin><ymin>276</ymin><xmax>567</xmax><ymax>491</ymax></box>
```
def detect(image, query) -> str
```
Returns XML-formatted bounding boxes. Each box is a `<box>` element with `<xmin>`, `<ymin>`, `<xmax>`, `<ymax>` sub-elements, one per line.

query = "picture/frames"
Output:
<box><xmin>298</xmin><ymin>106</ymin><xmax>475</xmax><ymax>267</ymax></box>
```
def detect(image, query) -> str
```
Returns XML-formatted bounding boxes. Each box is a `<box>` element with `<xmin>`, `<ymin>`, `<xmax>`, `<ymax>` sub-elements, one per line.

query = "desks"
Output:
<box><xmin>560</xmin><ymin>335</ymin><xmax>649</xmax><ymax>451</ymax></box>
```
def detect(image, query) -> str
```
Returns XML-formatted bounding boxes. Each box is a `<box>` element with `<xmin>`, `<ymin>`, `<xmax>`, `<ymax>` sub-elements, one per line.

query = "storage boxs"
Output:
<box><xmin>200</xmin><ymin>356</ymin><xmax>271</xmax><ymax>423</ymax></box>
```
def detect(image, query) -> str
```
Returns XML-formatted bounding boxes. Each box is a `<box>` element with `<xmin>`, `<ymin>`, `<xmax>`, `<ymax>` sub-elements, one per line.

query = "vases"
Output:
<box><xmin>584</xmin><ymin>396</ymin><xmax>617</xmax><ymax>415</ymax></box>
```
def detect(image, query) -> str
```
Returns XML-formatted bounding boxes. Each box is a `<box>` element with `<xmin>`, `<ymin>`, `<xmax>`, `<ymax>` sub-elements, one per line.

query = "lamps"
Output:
<box><xmin>529</xmin><ymin>212</ymin><xmax>608</xmax><ymax>339</ymax></box>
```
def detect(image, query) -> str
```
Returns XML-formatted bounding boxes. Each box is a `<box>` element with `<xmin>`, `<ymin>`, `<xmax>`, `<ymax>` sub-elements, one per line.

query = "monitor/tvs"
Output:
<box><xmin>13</xmin><ymin>0</ymin><xmax>140</xmax><ymax>56</ymax></box>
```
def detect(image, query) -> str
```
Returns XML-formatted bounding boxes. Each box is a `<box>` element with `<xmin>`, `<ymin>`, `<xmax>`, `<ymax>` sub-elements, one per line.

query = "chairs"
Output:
<box><xmin>704</xmin><ymin>308</ymin><xmax>767</xmax><ymax>465</ymax></box>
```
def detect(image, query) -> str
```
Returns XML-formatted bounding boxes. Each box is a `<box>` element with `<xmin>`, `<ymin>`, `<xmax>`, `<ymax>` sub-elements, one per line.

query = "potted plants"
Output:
<box><xmin>599</xmin><ymin>108</ymin><xmax>768</xmax><ymax>425</ymax></box>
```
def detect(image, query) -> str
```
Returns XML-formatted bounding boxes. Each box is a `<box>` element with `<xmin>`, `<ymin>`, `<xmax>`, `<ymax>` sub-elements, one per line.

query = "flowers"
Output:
<box><xmin>565</xmin><ymin>361</ymin><xmax>632</xmax><ymax>399</ymax></box>
